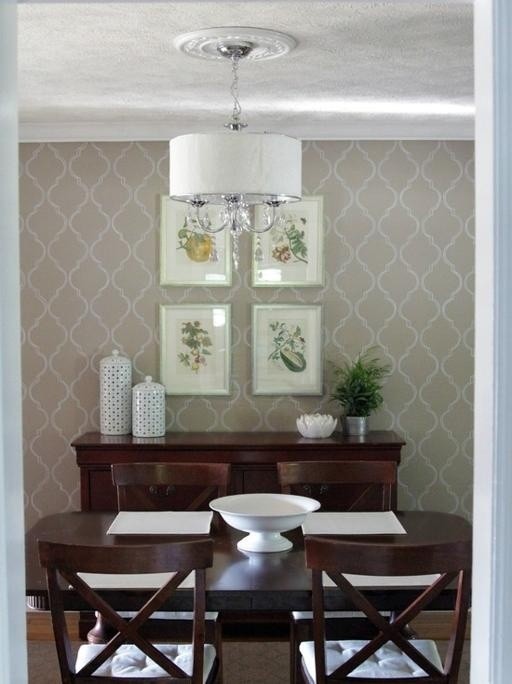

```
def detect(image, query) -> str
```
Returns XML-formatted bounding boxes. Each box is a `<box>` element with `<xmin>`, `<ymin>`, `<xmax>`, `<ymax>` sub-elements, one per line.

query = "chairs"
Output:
<box><xmin>292</xmin><ymin>536</ymin><xmax>473</xmax><ymax>682</ymax></box>
<box><xmin>110</xmin><ymin>463</ymin><xmax>228</xmax><ymax>661</ymax></box>
<box><xmin>40</xmin><ymin>537</ymin><xmax>223</xmax><ymax>684</ymax></box>
<box><xmin>278</xmin><ymin>461</ymin><xmax>398</xmax><ymax>679</ymax></box>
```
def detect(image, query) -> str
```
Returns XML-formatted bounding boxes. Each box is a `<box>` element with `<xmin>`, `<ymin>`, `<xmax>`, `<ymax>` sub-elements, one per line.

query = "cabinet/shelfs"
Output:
<box><xmin>72</xmin><ymin>430</ymin><xmax>407</xmax><ymax>643</ymax></box>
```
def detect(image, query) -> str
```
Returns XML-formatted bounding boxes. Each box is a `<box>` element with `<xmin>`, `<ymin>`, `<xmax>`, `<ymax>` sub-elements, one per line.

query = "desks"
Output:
<box><xmin>26</xmin><ymin>510</ymin><xmax>472</xmax><ymax>625</ymax></box>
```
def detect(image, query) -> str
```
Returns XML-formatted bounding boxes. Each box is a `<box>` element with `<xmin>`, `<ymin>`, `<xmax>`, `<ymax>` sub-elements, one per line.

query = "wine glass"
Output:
<box><xmin>208</xmin><ymin>492</ymin><xmax>322</xmax><ymax>554</ymax></box>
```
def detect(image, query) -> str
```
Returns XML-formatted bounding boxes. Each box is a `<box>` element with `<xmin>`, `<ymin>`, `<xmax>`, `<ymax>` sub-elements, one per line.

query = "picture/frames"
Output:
<box><xmin>155</xmin><ymin>192</ymin><xmax>325</xmax><ymax>397</ymax></box>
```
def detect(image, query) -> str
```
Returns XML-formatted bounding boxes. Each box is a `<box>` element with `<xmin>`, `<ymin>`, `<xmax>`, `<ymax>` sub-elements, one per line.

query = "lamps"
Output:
<box><xmin>166</xmin><ymin>23</ymin><xmax>303</xmax><ymax>255</ymax></box>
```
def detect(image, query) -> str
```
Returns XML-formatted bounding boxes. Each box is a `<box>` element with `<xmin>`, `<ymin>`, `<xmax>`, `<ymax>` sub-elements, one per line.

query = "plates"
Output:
<box><xmin>301</xmin><ymin>510</ymin><xmax>408</xmax><ymax>537</ymax></box>
<box><xmin>106</xmin><ymin>510</ymin><xmax>214</xmax><ymax>536</ymax></box>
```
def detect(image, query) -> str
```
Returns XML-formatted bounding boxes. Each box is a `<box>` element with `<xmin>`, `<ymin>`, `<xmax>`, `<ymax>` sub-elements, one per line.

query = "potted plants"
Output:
<box><xmin>321</xmin><ymin>343</ymin><xmax>392</xmax><ymax>437</ymax></box>
